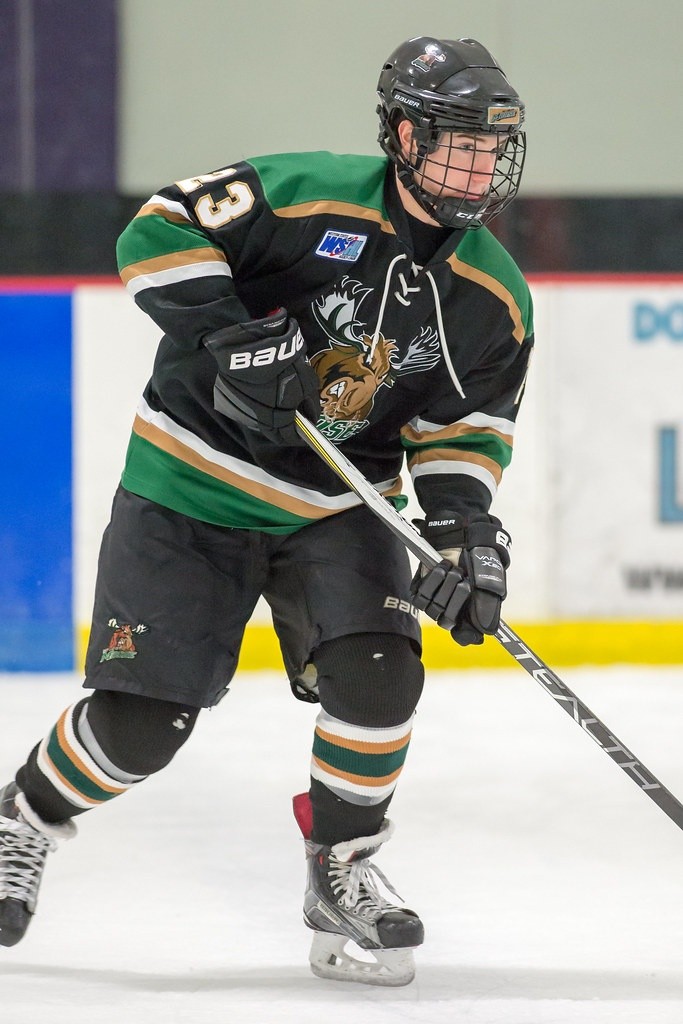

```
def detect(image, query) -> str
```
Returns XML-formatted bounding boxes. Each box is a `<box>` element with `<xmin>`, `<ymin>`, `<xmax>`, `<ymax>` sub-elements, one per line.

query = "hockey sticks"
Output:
<box><xmin>294</xmin><ymin>408</ymin><xmax>682</xmax><ymax>836</ymax></box>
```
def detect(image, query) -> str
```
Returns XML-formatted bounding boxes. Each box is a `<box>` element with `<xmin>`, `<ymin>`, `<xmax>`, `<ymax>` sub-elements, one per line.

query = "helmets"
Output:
<box><xmin>377</xmin><ymin>35</ymin><xmax>527</xmax><ymax>228</ymax></box>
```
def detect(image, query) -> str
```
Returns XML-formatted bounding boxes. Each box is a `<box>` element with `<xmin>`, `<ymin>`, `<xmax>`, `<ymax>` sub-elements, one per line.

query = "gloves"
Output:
<box><xmin>200</xmin><ymin>307</ymin><xmax>322</xmax><ymax>447</ymax></box>
<box><xmin>410</xmin><ymin>506</ymin><xmax>512</xmax><ymax>646</ymax></box>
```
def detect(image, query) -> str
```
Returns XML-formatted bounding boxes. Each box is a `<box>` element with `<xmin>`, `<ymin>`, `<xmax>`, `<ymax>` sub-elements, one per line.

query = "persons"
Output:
<box><xmin>0</xmin><ymin>38</ymin><xmax>533</xmax><ymax>985</ymax></box>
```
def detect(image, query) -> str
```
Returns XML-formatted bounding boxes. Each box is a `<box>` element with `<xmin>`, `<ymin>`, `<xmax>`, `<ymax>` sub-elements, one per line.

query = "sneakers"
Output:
<box><xmin>292</xmin><ymin>791</ymin><xmax>424</xmax><ymax>987</ymax></box>
<box><xmin>0</xmin><ymin>781</ymin><xmax>78</xmax><ymax>946</ymax></box>
